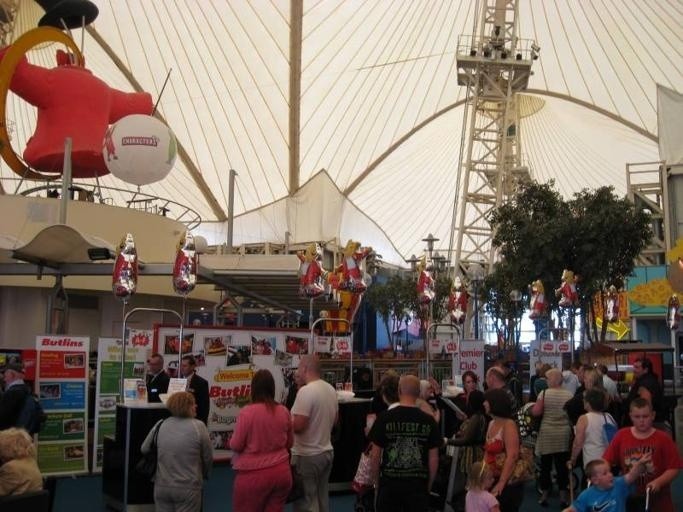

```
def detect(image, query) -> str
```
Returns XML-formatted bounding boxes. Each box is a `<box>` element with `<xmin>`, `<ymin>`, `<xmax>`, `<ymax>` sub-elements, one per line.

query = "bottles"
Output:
<box><xmin>136</xmin><ymin>383</ymin><xmax>147</xmax><ymax>407</ymax></box>
<box><xmin>318</xmin><ymin>345</ymin><xmax>425</xmax><ymax>359</ymax></box>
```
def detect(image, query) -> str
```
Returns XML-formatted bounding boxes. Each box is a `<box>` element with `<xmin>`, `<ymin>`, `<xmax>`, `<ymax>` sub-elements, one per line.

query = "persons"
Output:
<box><xmin>40</xmin><ymin>385</ymin><xmax>59</xmax><ymax>398</ymax></box>
<box><xmin>2</xmin><ymin>354</ymin><xmax>46</xmax><ymax>497</ymax></box>
<box><xmin>229</xmin><ymin>353</ymin><xmax>339</xmax><ymax>511</ymax></box>
<box><xmin>351</xmin><ymin>362</ymin><xmax>683</xmax><ymax>511</ymax></box>
<box><xmin>140</xmin><ymin>352</ymin><xmax>214</xmax><ymax>511</ymax></box>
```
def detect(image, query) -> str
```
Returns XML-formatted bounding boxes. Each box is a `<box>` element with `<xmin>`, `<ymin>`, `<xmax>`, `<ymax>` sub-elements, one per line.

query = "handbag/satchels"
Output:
<box><xmin>494</xmin><ymin>446</ymin><xmax>541</xmax><ymax>488</ymax></box>
<box><xmin>135</xmin><ymin>442</ymin><xmax>158</xmax><ymax>483</ymax></box>
<box><xmin>351</xmin><ymin>445</ymin><xmax>382</xmax><ymax>497</ymax></box>
<box><xmin>532</xmin><ymin>413</ymin><xmax>544</xmax><ymax>433</ymax></box>
<box><xmin>603</xmin><ymin>423</ymin><xmax>623</xmax><ymax>467</ymax></box>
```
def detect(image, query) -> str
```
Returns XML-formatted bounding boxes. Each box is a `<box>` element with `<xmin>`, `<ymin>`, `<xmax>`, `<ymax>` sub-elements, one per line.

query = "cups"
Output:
<box><xmin>620</xmin><ymin>392</ymin><xmax>628</xmax><ymax>399</ymax></box>
<box><xmin>344</xmin><ymin>382</ymin><xmax>351</xmax><ymax>392</ymax></box>
<box><xmin>335</xmin><ymin>382</ymin><xmax>342</xmax><ymax>390</ymax></box>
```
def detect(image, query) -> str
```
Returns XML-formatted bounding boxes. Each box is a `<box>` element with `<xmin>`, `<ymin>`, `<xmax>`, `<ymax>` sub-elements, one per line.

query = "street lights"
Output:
<box><xmin>466</xmin><ymin>263</ymin><xmax>486</xmax><ymax>341</ymax></box>
<box><xmin>510</xmin><ymin>289</ymin><xmax>520</xmax><ymax>348</ymax></box>
<box><xmin>406</xmin><ymin>232</ymin><xmax>450</xmax><ymax>351</ymax></box>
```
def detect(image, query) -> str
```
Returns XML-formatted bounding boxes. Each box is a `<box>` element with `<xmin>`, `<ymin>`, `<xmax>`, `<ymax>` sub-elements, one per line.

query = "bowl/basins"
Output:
<box><xmin>158</xmin><ymin>393</ymin><xmax>169</xmax><ymax>403</ymax></box>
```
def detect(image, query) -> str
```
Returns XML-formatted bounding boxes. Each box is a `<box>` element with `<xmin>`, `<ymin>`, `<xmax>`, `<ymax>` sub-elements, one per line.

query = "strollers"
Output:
<box><xmin>587</xmin><ymin>479</ymin><xmax>651</xmax><ymax>511</ymax></box>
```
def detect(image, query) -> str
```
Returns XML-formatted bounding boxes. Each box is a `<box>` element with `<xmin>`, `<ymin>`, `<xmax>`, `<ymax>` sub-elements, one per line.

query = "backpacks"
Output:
<box><xmin>10</xmin><ymin>386</ymin><xmax>43</xmax><ymax>434</ymax></box>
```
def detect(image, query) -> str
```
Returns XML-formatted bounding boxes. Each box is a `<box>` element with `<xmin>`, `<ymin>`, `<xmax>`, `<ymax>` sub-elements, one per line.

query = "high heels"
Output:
<box><xmin>559</xmin><ymin>494</ymin><xmax>569</xmax><ymax>509</ymax></box>
<box><xmin>538</xmin><ymin>495</ymin><xmax>550</xmax><ymax>506</ymax></box>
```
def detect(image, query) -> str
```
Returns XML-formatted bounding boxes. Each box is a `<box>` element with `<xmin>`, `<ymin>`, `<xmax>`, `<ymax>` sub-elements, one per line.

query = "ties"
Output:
<box><xmin>146</xmin><ymin>376</ymin><xmax>154</xmax><ymax>386</ymax></box>
